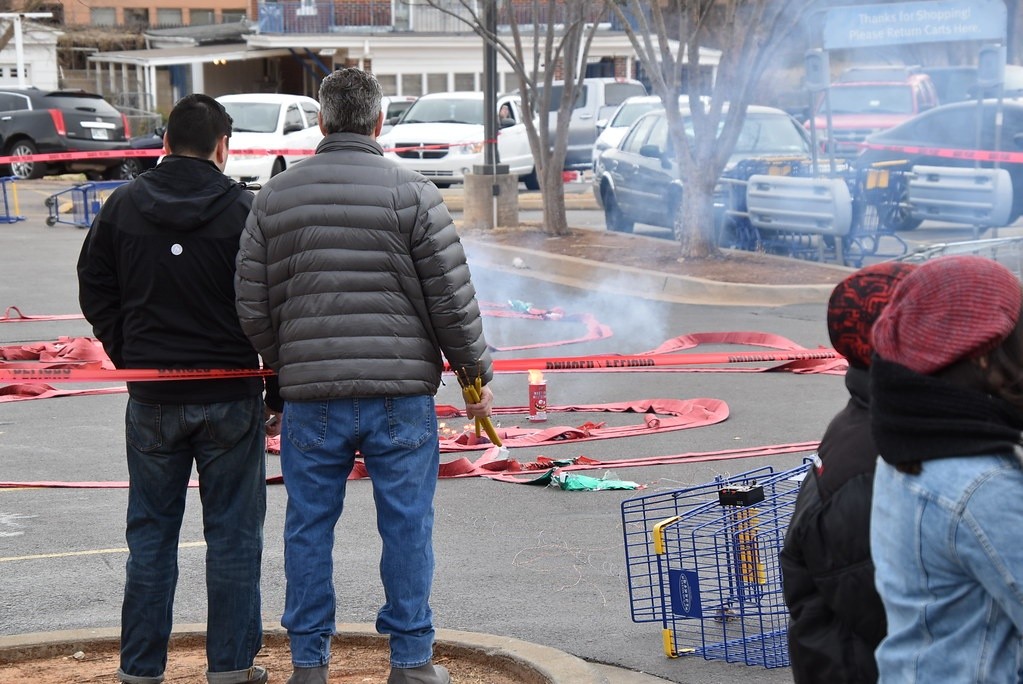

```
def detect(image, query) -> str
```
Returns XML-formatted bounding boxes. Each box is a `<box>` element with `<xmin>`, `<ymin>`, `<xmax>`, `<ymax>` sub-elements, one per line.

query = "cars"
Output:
<box><xmin>379</xmin><ymin>91</ymin><xmax>549</xmax><ymax>194</ymax></box>
<box><xmin>921</xmin><ymin>63</ymin><xmax>1022</xmax><ymax>108</ymax></box>
<box><xmin>115</xmin><ymin>125</ymin><xmax>165</xmax><ymax>181</ymax></box>
<box><xmin>376</xmin><ymin>95</ymin><xmax>417</xmax><ymax>136</ymax></box>
<box><xmin>157</xmin><ymin>93</ymin><xmax>325</xmax><ymax>195</ymax></box>
<box><xmin>595</xmin><ymin>107</ymin><xmax>868</xmax><ymax>255</ymax></box>
<box><xmin>860</xmin><ymin>95</ymin><xmax>1023</xmax><ymax>232</ymax></box>
<box><xmin>592</xmin><ymin>92</ymin><xmax>715</xmax><ymax>208</ymax></box>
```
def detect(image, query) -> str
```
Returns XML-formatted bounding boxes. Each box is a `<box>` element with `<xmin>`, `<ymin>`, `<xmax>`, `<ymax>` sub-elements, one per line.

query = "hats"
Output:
<box><xmin>829</xmin><ymin>263</ymin><xmax>918</xmax><ymax>369</ymax></box>
<box><xmin>869</xmin><ymin>252</ymin><xmax>1023</xmax><ymax>375</ymax></box>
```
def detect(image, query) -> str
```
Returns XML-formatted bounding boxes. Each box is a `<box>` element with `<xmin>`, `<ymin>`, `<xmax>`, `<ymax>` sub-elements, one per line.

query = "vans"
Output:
<box><xmin>507</xmin><ymin>78</ymin><xmax>648</xmax><ymax>171</ymax></box>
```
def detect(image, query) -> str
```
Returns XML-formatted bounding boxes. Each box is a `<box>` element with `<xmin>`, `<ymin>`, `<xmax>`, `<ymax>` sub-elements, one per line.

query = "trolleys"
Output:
<box><xmin>0</xmin><ymin>175</ymin><xmax>25</xmax><ymax>225</ymax></box>
<box><xmin>620</xmin><ymin>452</ymin><xmax>817</xmax><ymax>670</ymax></box>
<box><xmin>44</xmin><ymin>178</ymin><xmax>126</xmax><ymax>230</ymax></box>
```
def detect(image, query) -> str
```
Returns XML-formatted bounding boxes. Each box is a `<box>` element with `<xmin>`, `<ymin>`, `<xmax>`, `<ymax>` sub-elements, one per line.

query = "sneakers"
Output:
<box><xmin>388</xmin><ymin>662</ymin><xmax>450</xmax><ymax>684</ymax></box>
<box><xmin>237</xmin><ymin>664</ymin><xmax>268</xmax><ymax>684</ymax></box>
<box><xmin>285</xmin><ymin>665</ymin><xmax>328</xmax><ymax>684</ymax></box>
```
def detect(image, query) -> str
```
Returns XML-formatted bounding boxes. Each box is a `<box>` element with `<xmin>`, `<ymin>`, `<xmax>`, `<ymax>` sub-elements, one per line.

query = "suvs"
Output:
<box><xmin>804</xmin><ymin>65</ymin><xmax>943</xmax><ymax>160</ymax></box>
<box><xmin>1</xmin><ymin>87</ymin><xmax>130</xmax><ymax>180</ymax></box>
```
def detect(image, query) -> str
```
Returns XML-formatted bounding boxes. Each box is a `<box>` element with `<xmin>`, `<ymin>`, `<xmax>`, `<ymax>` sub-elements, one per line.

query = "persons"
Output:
<box><xmin>499</xmin><ymin>104</ymin><xmax>514</xmax><ymax>127</ymax></box>
<box><xmin>235</xmin><ymin>67</ymin><xmax>493</xmax><ymax>683</ymax></box>
<box><xmin>76</xmin><ymin>94</ymin><xmax>285</xmax><ymax>683</ymax></box>
<box><xmin>782</xmin><ymin>262</ymin><xmax>917</xmax><ymax>684</ymax></box>
<box><xmin>871</xmin><ymin>253</ymin><xmax>1023</xmax><ymax>682</ymax></box>
